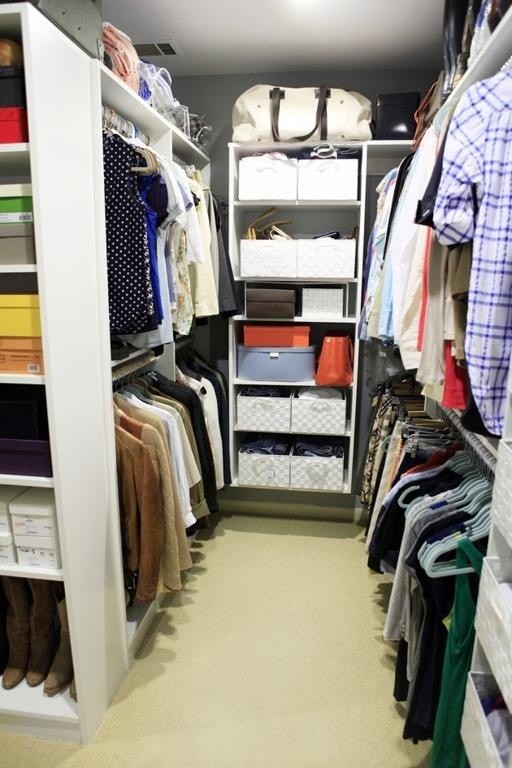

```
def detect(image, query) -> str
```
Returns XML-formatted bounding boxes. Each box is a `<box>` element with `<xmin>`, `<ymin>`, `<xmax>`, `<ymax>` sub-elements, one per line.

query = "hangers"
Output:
<box><xmin>110</xmin><ymin>336</ymin><xmax>162</xmax><ymax>410</ymax></box>
<box><xmin>101</xmin><ymin>104</ymin><xmax>222</xmax><ymax>183</ymax></box>
<box><xmin>389</xmin><ymin>379</ymin><xmax>499</xmax><ymax>578</ymax></box>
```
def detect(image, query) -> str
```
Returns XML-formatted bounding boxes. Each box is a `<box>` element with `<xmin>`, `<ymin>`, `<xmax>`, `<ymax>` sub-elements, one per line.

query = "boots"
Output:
<box><xmin>2</xmin><ymin>577</ymin><xmax>77</xmax><ymax>699</ymax></box>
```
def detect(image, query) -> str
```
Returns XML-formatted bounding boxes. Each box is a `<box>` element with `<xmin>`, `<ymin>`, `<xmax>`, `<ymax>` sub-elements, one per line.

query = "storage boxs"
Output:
<box><xmin>236</xmin><ymin>152</ymin><xmax>358</xmax><ymax>491</ymax></box>
<box><xmin>0</xmin><ymin>108</ymin><xmax>60</xmax><ymax>571</ymax></box>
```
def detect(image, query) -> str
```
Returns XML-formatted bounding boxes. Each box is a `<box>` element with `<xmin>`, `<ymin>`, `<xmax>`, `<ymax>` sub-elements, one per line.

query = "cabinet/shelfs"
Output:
<box><xmin>0</xmin><ymin>2</ymin><xmax>109</xmax><ymax>748</ymax></box>
<box><xmin>458</xmin><ymin>386</ymin><xmax>512</xmax><ymax>768</ymax></box>
<box><xmin>219</xmin><ymin>142</ymin><xmax>366</xmax><ymax>525</ymax></box>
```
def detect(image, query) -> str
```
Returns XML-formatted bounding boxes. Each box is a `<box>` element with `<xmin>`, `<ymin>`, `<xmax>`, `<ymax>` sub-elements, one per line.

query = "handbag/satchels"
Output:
<box><xmin>247</xmin><ymin>207</ymin><xmax>294</xmax><ymax>240</ymax></box>
<box><xmin>232</xmin><ymin>83</ymin><xmax>372</xmax><ymax>142</ymax></box>
<box><xmin>315</xmin><ymin>334</ymin><xmax>354</xmax><ymax>386</ymax></box>
